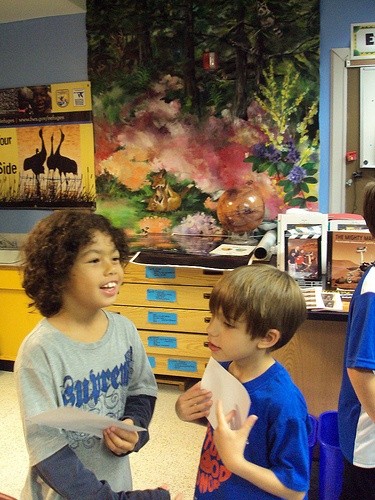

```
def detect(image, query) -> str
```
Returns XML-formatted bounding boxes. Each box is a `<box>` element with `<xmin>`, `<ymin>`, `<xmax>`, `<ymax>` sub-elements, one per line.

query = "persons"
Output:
<box><xmin>175</xmin><ymin>264</ymin><xmax>311</xmax><ymax>500</ymax></box>
<box><xmin>14</xmin><ymin>210</ymin><xmax>184</xmax><ymax>500</ymax></box>
<box><xmin>337</xmin><ymin>179</ymin><xmax>375</xmax><ymax>500</ymax></box>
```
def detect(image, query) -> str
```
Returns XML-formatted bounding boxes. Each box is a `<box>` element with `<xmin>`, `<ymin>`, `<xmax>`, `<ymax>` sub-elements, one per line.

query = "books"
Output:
<box><xmin>277</xmin><ymin>211</ymin><xmax>375</xmax><ymax>291</ymax></box>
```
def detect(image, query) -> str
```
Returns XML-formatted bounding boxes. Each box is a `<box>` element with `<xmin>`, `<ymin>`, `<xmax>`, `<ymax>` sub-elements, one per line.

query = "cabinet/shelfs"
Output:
<box><xmin>106</xmin><ymin>260</ymin><xmax>350</xmax><ymax>416</ymax></box>
